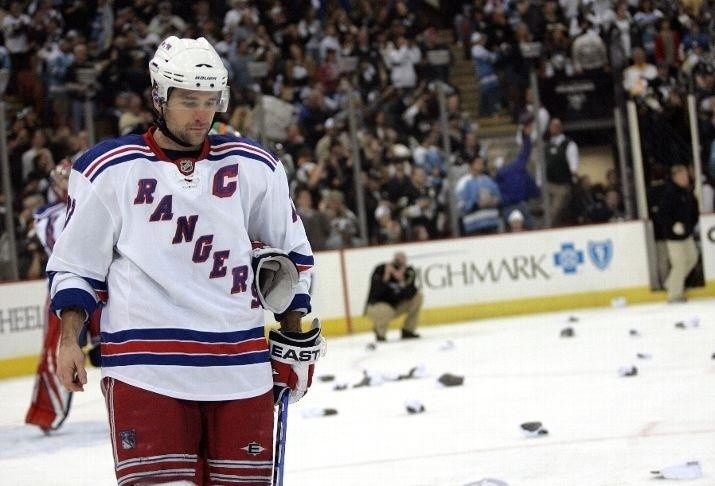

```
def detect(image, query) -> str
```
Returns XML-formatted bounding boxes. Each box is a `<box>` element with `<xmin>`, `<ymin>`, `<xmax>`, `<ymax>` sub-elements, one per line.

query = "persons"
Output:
<box><xmin>362</xmin><ymin>250</ymin><xmax>423</xmax><ymax>342</ymax></box>
<box><xmin>22</xmin><ymin>159</ymin><xmax>101</xmax><ymax>435</ymax></box>
<box><xmin>46</xmin><ymin>34</ymin><xmax>328</xmax><ymax>486</ymax></box>
<box><xmin>0</xmin><ymin>0</ymin><xmax>715</xmax><ymax>281</ymax></box>
<box><xmin>654</xmin><ymin>160</ymin><xmax>701</xmax><ymax>301</ymax></box>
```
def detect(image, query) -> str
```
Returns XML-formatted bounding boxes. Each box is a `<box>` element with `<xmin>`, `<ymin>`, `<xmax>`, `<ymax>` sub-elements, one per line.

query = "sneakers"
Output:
<box><xmin>376</xmin><ymin>331</ymin><xmax>420</xmax><ymax>341</ymax></box>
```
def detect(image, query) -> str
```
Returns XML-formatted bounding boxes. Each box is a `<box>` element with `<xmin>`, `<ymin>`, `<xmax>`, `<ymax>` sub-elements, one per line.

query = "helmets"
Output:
<box><xmin>147</xmin><ymin>36</ymin><xmax>230</xmax><ymax>104</ymax></box>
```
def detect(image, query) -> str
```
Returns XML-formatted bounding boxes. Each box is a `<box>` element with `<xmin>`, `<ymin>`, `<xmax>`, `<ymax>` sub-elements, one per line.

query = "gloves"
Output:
<box><xmin>248</xmin><ymin>239</ymin><xmax>300</xmax><ymax>314</ymax></box>
<box><xmin>271</xmin><ymin>317</ymin><xmax>325</xmax><ymax>404</ymax></box>
